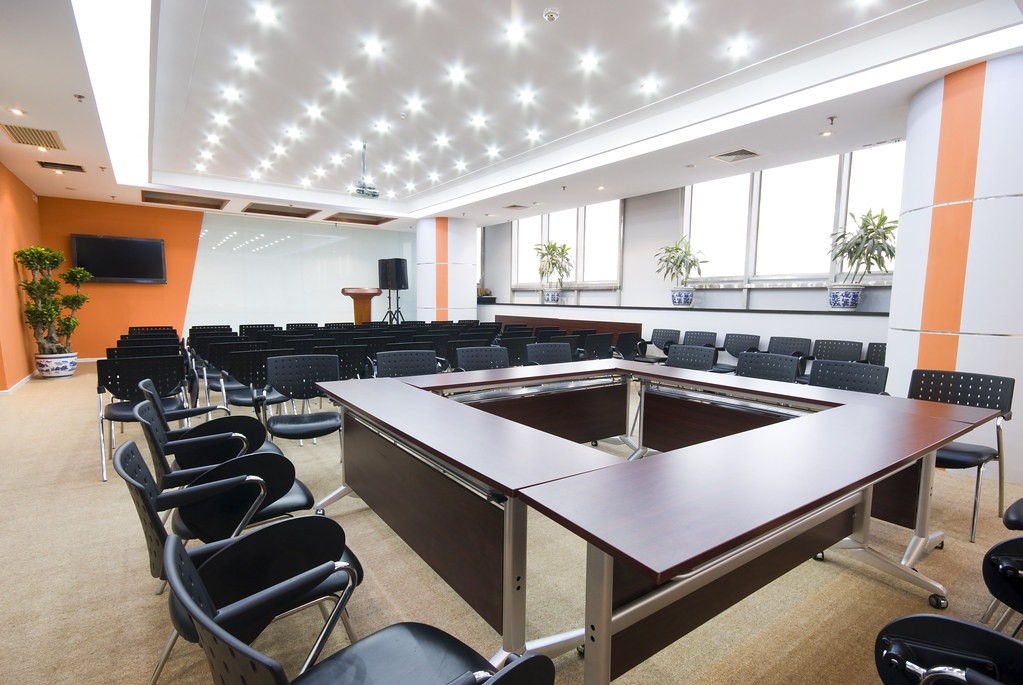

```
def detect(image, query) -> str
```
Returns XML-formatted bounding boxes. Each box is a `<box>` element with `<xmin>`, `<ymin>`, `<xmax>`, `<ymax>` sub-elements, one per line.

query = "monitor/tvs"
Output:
<box><xmin>71</xmin><ymin>234</ymin><xmax>167</xmax><ymax>285</ymax></box>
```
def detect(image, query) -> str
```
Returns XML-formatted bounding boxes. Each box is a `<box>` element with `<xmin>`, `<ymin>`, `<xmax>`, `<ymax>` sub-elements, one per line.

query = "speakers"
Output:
<box><xmin>379</xmin><ymin>259</ymin><xmax>409</xmax><ymax>290</ymax></box>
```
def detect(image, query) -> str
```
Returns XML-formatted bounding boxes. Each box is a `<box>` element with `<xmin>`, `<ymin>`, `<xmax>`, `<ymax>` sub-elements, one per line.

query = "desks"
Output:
<box><xmin>393</xmin><ymin>358</ymin><xmax>655</xmax><ymax>446</ymax></box>
<box><xmin>518</xmin><ymin>403</ymin><xmax>971</xmax><ymax>684</ymax></box>
<box><xmin>617</xmin><ymin>367</ymin><xmax>1002</xmax><ymax>572</ymax></box>
<box><xmin>313</xmin><ymin>376</ymin><xmax>630</xmax><ymax>669</ymax></box>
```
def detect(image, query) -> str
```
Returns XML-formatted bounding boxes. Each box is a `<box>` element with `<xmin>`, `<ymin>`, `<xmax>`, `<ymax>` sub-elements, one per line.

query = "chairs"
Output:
<box><xmin>96</xmin><ymin>320</ymin><xmax>1023</xmax><ymax>685</ymax></box>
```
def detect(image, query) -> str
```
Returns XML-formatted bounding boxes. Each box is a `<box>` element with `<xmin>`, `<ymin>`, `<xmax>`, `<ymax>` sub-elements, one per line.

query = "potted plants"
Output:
<box><xmin>14</xmin><ymin>246</ymin><xmax>94</xmax><ymax>378</ymax></box>
<box><xmin>534</xmin><ymin>241</ymin><xmax>573</xmax><ymax>303</ymax></box>
<box><xmin>826</xmin><ymin>208</ymin><xmax>898</xmax><ymax>311</ymax></box>
<box><xmin>654</xmin><ymin>236</ymin><xmax>708</xmax><ymax>306</ymax></box>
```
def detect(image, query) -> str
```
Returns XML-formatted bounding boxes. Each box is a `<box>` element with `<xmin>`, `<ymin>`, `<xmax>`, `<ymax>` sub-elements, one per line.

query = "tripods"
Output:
<box><xmin>382</xmin><ymin>289</ymin><xmax>405</xmax><ymax>326</ymax></box>
